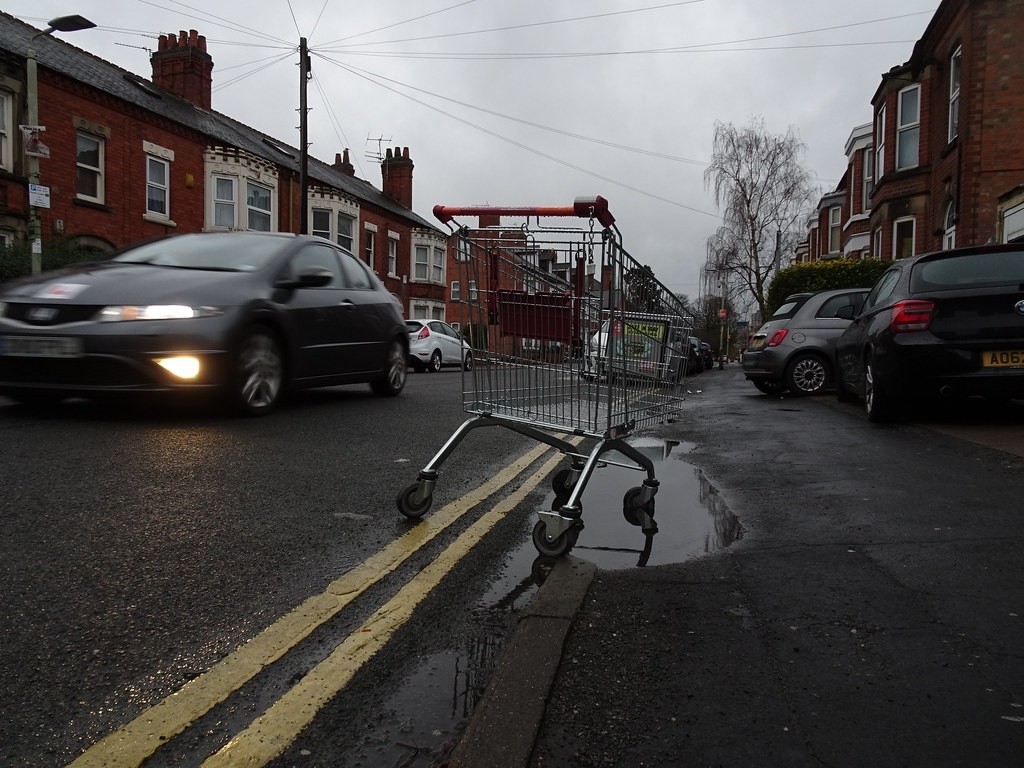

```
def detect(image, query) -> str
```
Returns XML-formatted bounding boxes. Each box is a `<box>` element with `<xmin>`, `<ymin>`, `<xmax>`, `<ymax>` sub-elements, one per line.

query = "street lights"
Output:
<box><xmin>21</xmin><ymin>14</ymin><xmax>97</xmax><ymax>275</ymax></box>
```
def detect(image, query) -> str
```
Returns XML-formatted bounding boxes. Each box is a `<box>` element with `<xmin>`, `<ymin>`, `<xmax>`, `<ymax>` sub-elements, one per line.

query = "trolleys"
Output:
<box><xmin>394</xmin><ymin>193</ymin><xmax>695</xmax><ymax>558</ymax></box>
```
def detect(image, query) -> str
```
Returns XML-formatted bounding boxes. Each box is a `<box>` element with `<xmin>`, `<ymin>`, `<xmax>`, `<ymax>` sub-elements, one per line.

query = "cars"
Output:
<box><xmin>583</xmin><ymin>316</ymin><xmax>611</xmax><ymax>381</ymax></box>
<box><xmin>701</xmin><ymin>342</ymin><xmax>714</xmax><ymax>370</ymax></box>
<box><xmin>0</xmin><ymin>227</ymin><xmax>410</xmax><ymax>418</ymax></box>
<box><xmin>687</xmin><ymin>336</ymin><xmax>707</xmax><ymax>376</ymax></box>
<box><xmin>741</xmin><ymin>287</ymin><xmax>874</xmax><ymax>395</ymax></box>
<box><xmin>670</xmin><ymin>332</ymin><xmax>698</xmax><ymax>376</ymax></box>
<box><xmin>404</xmin><ymin>318</ymin><xmax>474</xmax><ymax>372</ymax></box>
<box><xmin>834</xmin><ymin>241</ymin><xmax>1024</xmax><ymax>424</ymax></box>
